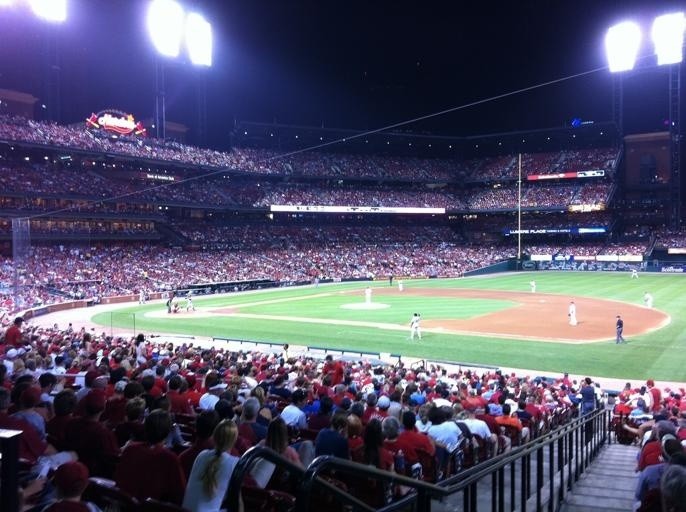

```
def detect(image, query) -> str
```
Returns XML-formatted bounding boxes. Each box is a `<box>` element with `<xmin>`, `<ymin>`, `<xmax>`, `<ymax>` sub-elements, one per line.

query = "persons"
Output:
<box><xmin>616</xmin><ymin>315</ymin><xmax>626</xmax><ymax>343</ymax></box>
<box><xmin>465</xmin><ymin>150</ymin><xmax>685</xmax><ymax>274</ymax></box>
<box><xmin>630</xmin><ymin>268</ymin><xmax>640</xmax><ymax>279</ymax></box>
<box><xmin>196</xmin><ymin>146</ymin><xmax>463</xmax><ymax>284</ymax></box>
<box><xmin>2</xmin><ymin>317</ymin><xmax>686</xmax><ymax>512</ymax></box>
<box><xmin>644</xmin><ymin>291</ymin><xmax>654</xmax><ymax>310</ymax></box>
<box><xmin>529</xmin><ymin>278</ymin><xmax>537</xmax><ymax>294</ymax></box>
<box><xmin>2</xmin><ymin>113</ymin><xmax>196</xmax><ymax>325</ymax></box>
<box><xmin>364</xmin><ymin>286</ymin><xmax>374</xmax><ymax>307</ymax></box>
<box><xmin>397</xmin><ymin>277</ymin><xmax>404</xmax><ymax>292</ymax></box>
<box><xmin>410</xmin><ymin>313</ymin><xmax>422</xmax><ymax>341</ymax></box>
<box><xmin>567</xmin><ymin>300</ymin><xmax>579</xmax><ymax>326</ymax></box>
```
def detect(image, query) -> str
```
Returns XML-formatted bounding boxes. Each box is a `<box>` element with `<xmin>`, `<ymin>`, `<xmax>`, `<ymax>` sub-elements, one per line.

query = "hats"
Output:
<box><xmin>661</xmin><ymin>434</ymin><xmax>677</xmax><ymax>461</ymax></box>
<box><xmin>463</xmin><ymin>397</ymin><xmax>485</xmax><ymax>410</ymax></box>
<box><xmin>6</xmin><ymin>347</ymin><xmax>30</xmax><ymax>360</ymax></box>
<box><xmin>84</xmin><ymin>390</ymin><xmax>105</xmax><ymax>414</ymax></box>
<box><xmin>56</xmin><ymin>462</ymin><xmax>90</xmax><ymax>496</ymax></box>
<box><xmin>15</xmin><ymin>318</ymin><xmax>25</xmax><ymax>323</ymax></box>
<box><xmin>20</xmin><ymin>387</ymin><xmax>41</xmax><ymax>407</ymax></box>
<box><xmin>115</xmin><ymin>381</ymin><xmax>127</xmax><ymax>393</ymax></box>
<box><xmin>378</xmin><ymin>396</ymin><xmax>390</xmax><ymax>409</ymax></box>
<box><xmin>271</xmin><ymin>374</ymin><xmax>284</xmax><ymax>385</ymax></box>
<box><xmin>162</xmin><ymin>356</ymin><xmax>200</xmax><ymax>383</ymax></box>
<box><xmin>209</xmin><ymin>382</ymin><xmax>228</xmax><ymax>390</ymax></box>
<box><xmin>619</xmin><ymin>380</ymin><xmax>654</xmax><ymax>410</ymax></box>
